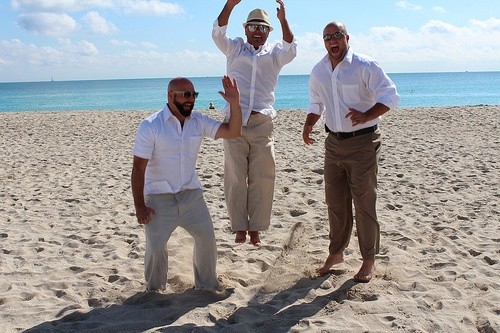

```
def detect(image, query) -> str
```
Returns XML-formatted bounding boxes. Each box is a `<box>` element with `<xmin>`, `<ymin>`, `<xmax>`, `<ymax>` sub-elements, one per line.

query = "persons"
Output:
<box><xmin>209</xmin><ymin>102</ymin><xmax>215</xmax><ymax>109</ymax></box>
<box><xmin>131</xmin><ymin>77</ymin><xmax>242</xmax><ymax>290</ymax></box>
<box><xmin>303</xmin><ymin>22</ymin><xmax>399</xmax><ymax>281</ymax></box>
<box><xmin>213</xmin><ymin>0</ymin><xmax>297</xmax><ymax>244</ymax></box>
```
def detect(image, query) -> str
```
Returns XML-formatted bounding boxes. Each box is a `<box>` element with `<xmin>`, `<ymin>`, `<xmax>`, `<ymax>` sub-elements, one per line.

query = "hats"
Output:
<box><xmin>242</xmin><ymin>8</ymin><xmax>274</xmax><ymax>32</ymax></box>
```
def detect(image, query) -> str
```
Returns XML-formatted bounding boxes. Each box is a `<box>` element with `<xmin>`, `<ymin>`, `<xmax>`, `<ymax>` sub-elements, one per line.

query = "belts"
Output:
<box><xmin>330</xmin><ymin>124</ymin><xmax>377</xmax><ymax>139</ymax></box>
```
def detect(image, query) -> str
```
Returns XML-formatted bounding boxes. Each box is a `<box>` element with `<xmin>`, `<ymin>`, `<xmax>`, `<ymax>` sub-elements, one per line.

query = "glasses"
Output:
<box><xmin>246</xmin><ymin>25</ymin><xmax>269</xmax><ymax>33</ymax></box>
<box><xmin>323</xmin><ymin>32</ymin><xmax>347</xmax><ymax>41</ymax></box>
<box><xmin>174</xmin><ymin>91</ymin><xmax>199</xmax><ymax>99</ymax></box>
